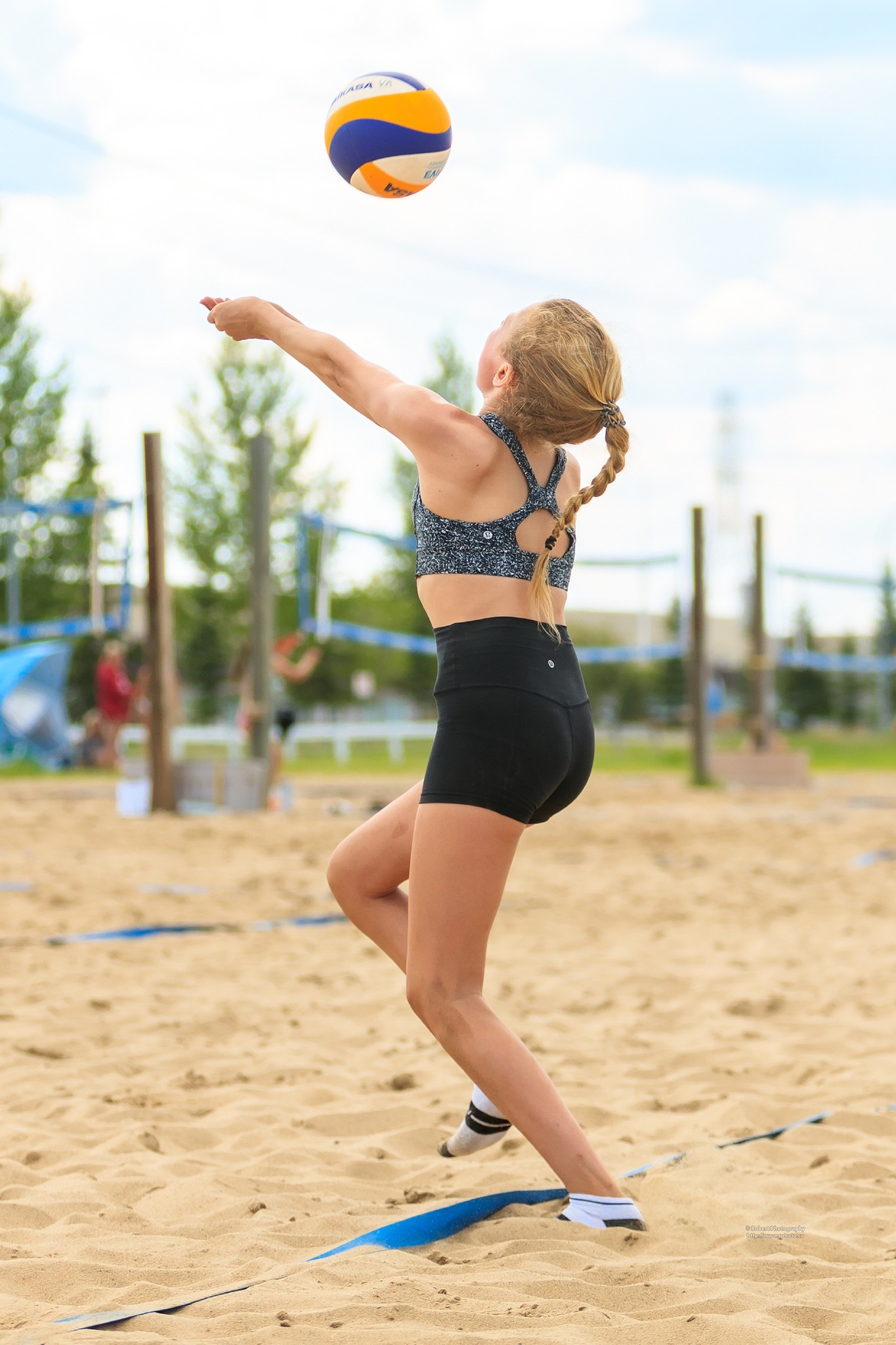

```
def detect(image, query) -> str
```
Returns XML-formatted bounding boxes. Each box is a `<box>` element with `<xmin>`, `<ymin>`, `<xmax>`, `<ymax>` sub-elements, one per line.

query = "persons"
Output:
<box><xmin>83</xmin><ymin>637</ymin><xmax>321</xmax><ymax>808</ymax></box>
<box><xmin>201</xmin><ymin>298</ymin><xmax>649</xmax><ymax>1234</ymax></box>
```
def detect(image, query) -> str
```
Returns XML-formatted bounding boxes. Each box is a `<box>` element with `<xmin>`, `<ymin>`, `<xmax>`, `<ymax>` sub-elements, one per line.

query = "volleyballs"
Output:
<box><xmin>324</xmin><ymin>71</ymin><xmax>452</xmax><ymax>198</ymax></box>
